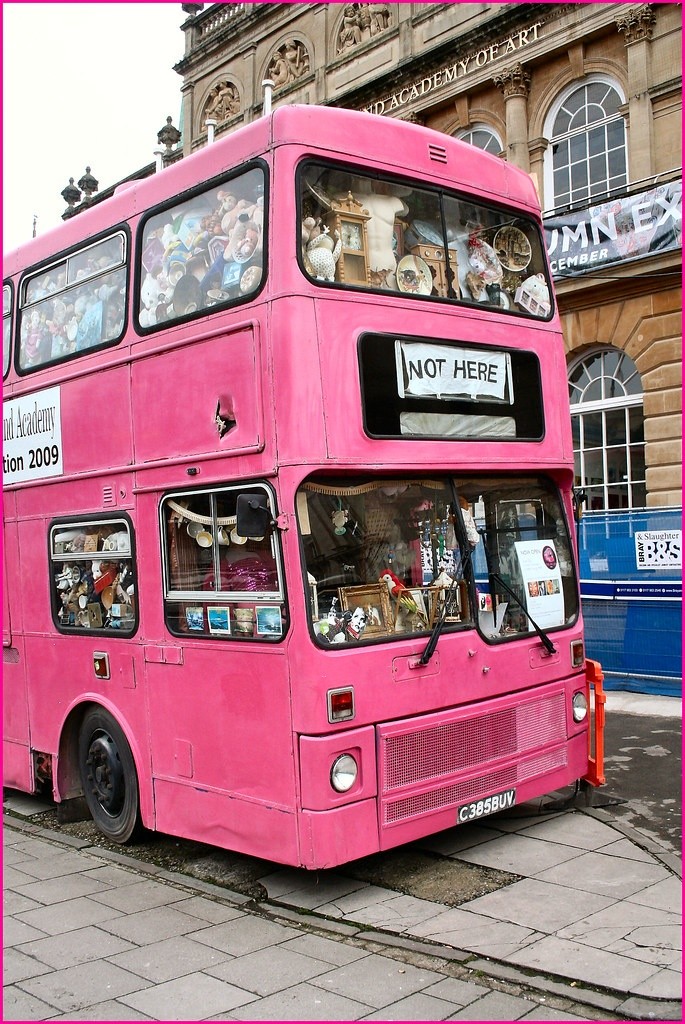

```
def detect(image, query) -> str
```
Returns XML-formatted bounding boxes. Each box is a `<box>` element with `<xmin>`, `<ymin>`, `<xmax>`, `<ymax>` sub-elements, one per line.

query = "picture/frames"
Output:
<box><xmin>394</xmin><ymin>585</ymin><xmax>440</xmax><ymax>632</ymax></box>
<box><xmin>339</xmin><ymin>582</ymin><xmax>393</xmax><ymax>642</ymax></box>
<box><xmin>428</xmin><ymin>586</ymin><xmax>461</xmax><ymax>623</ymax></box>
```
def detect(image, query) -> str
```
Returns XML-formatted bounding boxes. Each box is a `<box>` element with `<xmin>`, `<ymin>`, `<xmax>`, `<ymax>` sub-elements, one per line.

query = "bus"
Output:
<box><xmin>2</xmin><ymin>105</ymin><xmax>607</xmax><ymax>870</ymax></box>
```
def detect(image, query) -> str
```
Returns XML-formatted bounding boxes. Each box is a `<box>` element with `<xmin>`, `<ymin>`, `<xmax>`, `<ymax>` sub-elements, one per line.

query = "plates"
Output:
<box><xmin>397</xmin><ymin>255</ymin><xmax>432</xmax><ymax>295</ymax></box>
<box><xmin>493</xmin><ymin>227</ymin><xmax>532</xmax><ymax>272</ymax></box>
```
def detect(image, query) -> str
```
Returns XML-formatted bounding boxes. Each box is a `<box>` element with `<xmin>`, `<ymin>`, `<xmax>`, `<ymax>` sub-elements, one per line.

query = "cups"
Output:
<box><xmin>218</xmin><ymin>526</ymin><xmax>229</xmax><ymax>545</ymax></box>
<box><xmin>184</xmin><ymin>519</ymin><xmax>204</xmax><ymax>538</ymax></box>
<box><xmin>196</xmin><ymin>529</ymin><xmax>212</xmax><ymax>547</ymax></box>
<box><xmin>231</xmin><ymin>528</ymin><xmax>247</xmax><ymax>544</ymax></box>
<box><xmin>249</xmin><ymin>537</ymin><xmax>264</xmax><ymax>541</ymax></box>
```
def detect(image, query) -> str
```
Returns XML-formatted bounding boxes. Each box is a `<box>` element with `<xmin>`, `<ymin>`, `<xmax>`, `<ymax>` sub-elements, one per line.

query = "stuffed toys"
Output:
<box><xmin>139</xmin><ymin>189</ymin><xmax>265</xmax><ymax>329</ymax></box>
<box><xmin>302</xmin><ymin>216</ymin><xmax>341</xmax><ymax>282</ymax></box>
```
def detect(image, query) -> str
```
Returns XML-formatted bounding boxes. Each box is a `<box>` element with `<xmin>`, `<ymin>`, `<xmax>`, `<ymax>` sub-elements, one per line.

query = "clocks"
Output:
<box><xmin>321</xmin><ymin>188</ymin><xmax>371</xmax><ymax>286</ymax></box>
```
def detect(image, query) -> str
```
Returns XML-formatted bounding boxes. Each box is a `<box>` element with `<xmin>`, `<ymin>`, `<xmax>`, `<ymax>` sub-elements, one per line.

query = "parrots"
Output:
<box><xmin>378</xmin><ymin>570</ymin><xmax>429</xmax><ymax>627</ymax></box>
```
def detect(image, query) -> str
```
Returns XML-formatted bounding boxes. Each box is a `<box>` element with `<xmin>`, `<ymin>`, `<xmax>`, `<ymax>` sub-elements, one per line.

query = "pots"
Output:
<box><xmin>404</xmin><ymin>220</ymin><xmax>444</xmax><ymax>253</ymax></box>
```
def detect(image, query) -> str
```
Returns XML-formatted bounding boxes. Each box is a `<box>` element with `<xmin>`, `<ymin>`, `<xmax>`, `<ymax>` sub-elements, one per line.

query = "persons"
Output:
<box><xmin>338</xmin><ymin>3</ymin><xmax>387</xmax><ymax>54</ymax></box>
<box><xmin>205</xmin><ymin>79</ymin><xmax>233</xmax><ymax>119</ymax></box>
<box><xmin>269</xmin><ymin>39</ymin><xmax>304</xmax><ymax>86</ymax></box>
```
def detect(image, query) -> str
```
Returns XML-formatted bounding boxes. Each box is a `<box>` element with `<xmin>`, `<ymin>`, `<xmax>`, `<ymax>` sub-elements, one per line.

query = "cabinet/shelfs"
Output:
<box><xmin>413</xmin><ymin>244</ymin><xmax>459</xmax><ymax>300</ymax></box>
